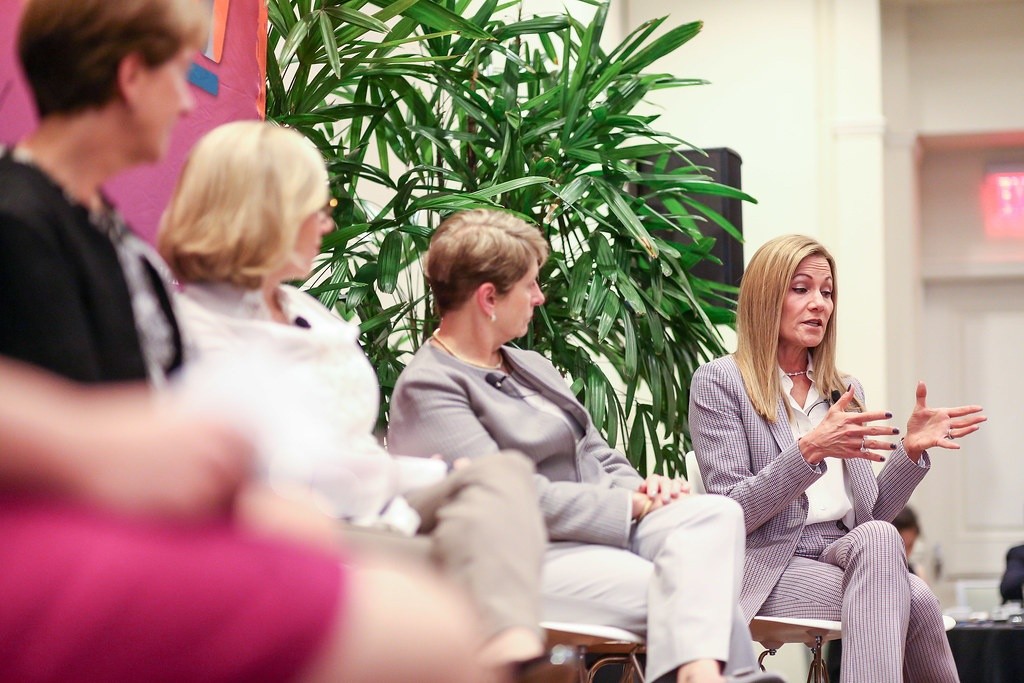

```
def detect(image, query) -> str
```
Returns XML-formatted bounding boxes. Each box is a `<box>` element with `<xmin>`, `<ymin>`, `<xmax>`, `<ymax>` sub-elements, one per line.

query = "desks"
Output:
<box><xmin>948</xmin><ymin>619</ymin><xmax>1024</xmax><ymax>683</ymax></box>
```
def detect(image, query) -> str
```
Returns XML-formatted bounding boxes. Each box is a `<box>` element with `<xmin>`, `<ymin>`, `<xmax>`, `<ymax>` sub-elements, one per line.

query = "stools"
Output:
<box><xmin>507</xmin><ymin>614</ymin><xmax>956</xmax><ymax>683</ymax></box>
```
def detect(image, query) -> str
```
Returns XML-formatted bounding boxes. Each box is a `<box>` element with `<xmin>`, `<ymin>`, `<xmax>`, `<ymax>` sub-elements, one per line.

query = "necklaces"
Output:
<box><xmin>785</xmin><ymin>371</ymin><xmax>807</xmax><ymax>376</ymax></box>
<box><xmin>434</xmin><ymin>329</ymin><xmax>503</xmax><ymax>370</ymax></box>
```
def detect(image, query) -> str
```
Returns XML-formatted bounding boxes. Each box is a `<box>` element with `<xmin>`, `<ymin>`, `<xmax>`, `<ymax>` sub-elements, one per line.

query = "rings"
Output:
<box><xmin>948</xmin><ymin>428</ymin><xmax>954</xmax><ymax>440</ymax></box>
<box><xmin>860</xmin><ymin>439</ymin><xmax>866</xmax><ymax>452</ymax></box>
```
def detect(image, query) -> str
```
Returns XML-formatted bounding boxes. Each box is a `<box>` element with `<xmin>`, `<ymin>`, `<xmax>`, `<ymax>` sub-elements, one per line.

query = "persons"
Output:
<box><xmin>388</xmin><ymin>208</ymin><xmax>783</xmax><ymax>683</ymax></box>
<box><xmin>2</xmin><ymin>0</ymin><xmax>568</xmax><ymax>683</ymax></box>
<box><xmin>689</xmin><ymin>233</ymin><xmax>989</xmax><ymax>683</ymax></box>
<box><xmin>890</xmin><ymin>506</ymin><xmax>924</xmax><ymax>577</ymax></box>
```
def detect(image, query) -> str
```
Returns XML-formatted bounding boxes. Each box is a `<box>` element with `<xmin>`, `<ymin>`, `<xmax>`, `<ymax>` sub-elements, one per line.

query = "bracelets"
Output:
<box><xmin>636</xmin><ymin>499</ymin><xmax>654</xmax><ymax>522</ymax></box>
